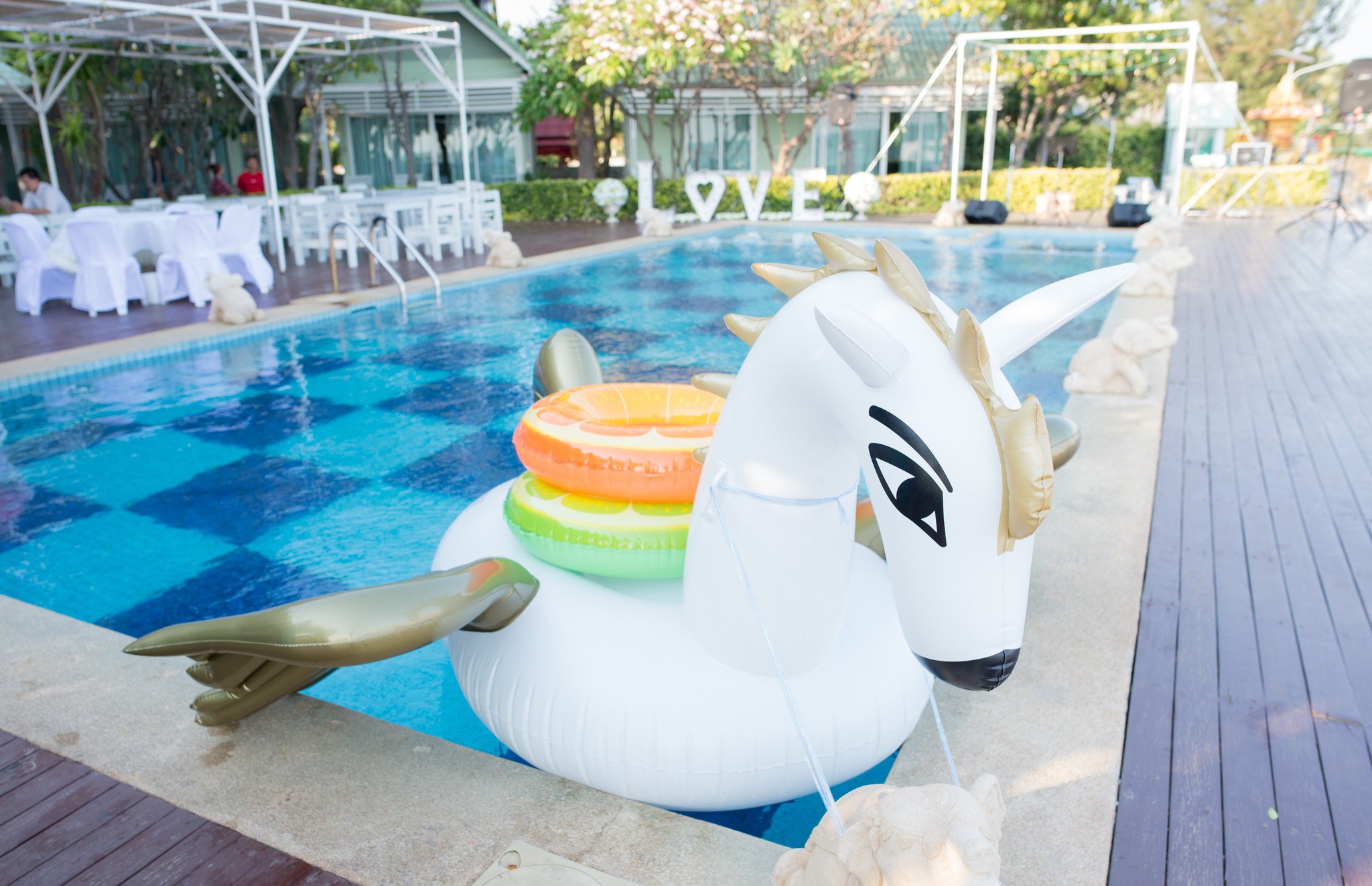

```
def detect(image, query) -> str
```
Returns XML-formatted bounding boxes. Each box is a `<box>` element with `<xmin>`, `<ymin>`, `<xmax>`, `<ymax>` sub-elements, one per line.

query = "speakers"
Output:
<box><xmin>1341</xmin><ymin>58</ymin><xmax>1372</xmax><ymax>113</ymax></box>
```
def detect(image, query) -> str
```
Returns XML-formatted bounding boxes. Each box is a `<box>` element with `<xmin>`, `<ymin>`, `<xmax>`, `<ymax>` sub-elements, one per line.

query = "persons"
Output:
<box><xmin>5</xmin><ymin>166</ymin><xmax>73</xmax><ymax>215</ymax></box>
<box><xmin>206</xmin><ymin>163</ymin><xmax>234</xmax><ymax>196</ymax></box>
<box><xmin>236</xmin><ymin>155</ymin><xmax>267</xmax><ymax>196</ymax></box>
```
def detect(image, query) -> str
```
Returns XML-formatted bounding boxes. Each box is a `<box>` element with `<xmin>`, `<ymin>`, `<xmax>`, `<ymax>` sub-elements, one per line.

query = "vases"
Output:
<box><xmin>602</xmin><ymin>205</ymin><xmax>622</xmax><ymax>225</ymax></box>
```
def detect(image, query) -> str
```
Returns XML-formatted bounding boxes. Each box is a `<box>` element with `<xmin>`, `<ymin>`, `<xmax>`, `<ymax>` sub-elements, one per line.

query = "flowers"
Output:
<box><xmin>594</xmin><ymin>177</ymin><xmax>629</xmax><ymax>206</ymax></box>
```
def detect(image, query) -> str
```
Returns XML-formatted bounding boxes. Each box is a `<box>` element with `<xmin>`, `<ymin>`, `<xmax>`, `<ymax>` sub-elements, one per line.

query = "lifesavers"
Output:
<box><xmin>510</xmin><ymin>380</ymin><xmax>729</xmax><ymax>506</ymax></box>
<box><xmin>500</xmin><ymin>467</ymin><xmax>695</xmax><ymax>581</ymax></box>
<box><xmin>122</xmin><ymin>230</ymin><xmax>1137</xmax><ymax>813</ymax></box>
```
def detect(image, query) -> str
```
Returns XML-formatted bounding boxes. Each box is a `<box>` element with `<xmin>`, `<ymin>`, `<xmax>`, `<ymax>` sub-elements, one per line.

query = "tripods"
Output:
<box><xmin>1278</xmin><ymin>112</ymin><xmax>1371</xmax><ymax>235</ymax></box>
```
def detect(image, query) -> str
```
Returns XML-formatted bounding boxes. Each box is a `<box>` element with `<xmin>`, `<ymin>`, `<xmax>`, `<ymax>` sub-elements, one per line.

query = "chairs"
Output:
<box><xmin>0</xmin><ymin>179</ymin><xmax>505</xmax><ymax>319</ymax></box>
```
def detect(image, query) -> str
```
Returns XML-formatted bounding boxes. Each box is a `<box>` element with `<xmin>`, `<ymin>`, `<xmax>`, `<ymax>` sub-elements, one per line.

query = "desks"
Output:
<box><xmin>360</xmin><ymin>191</ymin><xmax>458</xmax><ymax>260</ymax></box>
<box><xmin>39</xmin><ymin>212</ymin><xmax>218</xmax><ymax>260</ymax></box>
